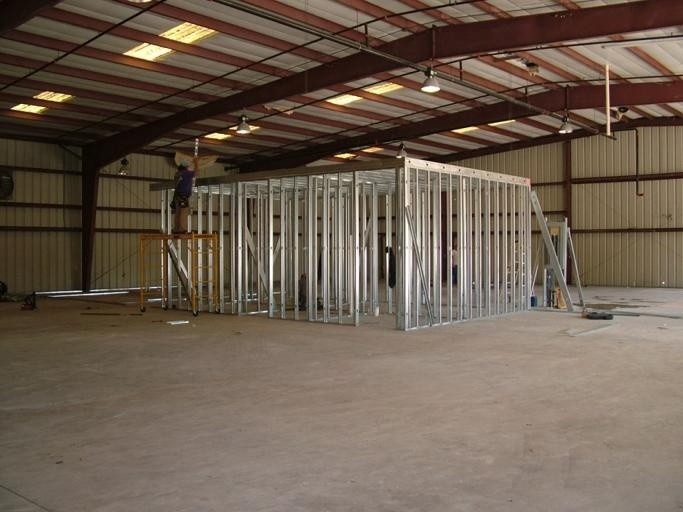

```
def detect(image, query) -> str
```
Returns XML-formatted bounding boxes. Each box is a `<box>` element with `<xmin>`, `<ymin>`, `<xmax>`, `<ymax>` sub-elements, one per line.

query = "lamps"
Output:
<box><xmin>421</xmin><ymin>67</ymin><xmax>441</xmax><ymax>92</ymax></box>
<box><xmin>118</xmin><ymin>159</ymin><xmax>129</xmax><ymax>175</ymax></box>
<box><xmin>396</xmin><ymin>138</ymin><xmax>408</xmax><ymax>159</ymax></box>
<box><xmin>558</xmin><ymin>110</ymin><xmax>573</xmax><ymax>134</ymax></box>
<box><xmin>236</xmin><ymin>108</ymin><xmax>250</xmax><ymax>134</ymax></box>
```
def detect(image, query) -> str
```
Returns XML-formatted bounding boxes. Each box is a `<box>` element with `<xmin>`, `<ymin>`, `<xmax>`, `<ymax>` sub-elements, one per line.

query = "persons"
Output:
<box><xmin>173</xmin><ymin>158</ymin><xmax>200</xmax><ymax>233</ymax></box>
<box><xmin>452</xmin><ymin>245</ymin><xmax>457</xmax><ymax>284</ymax></box>
